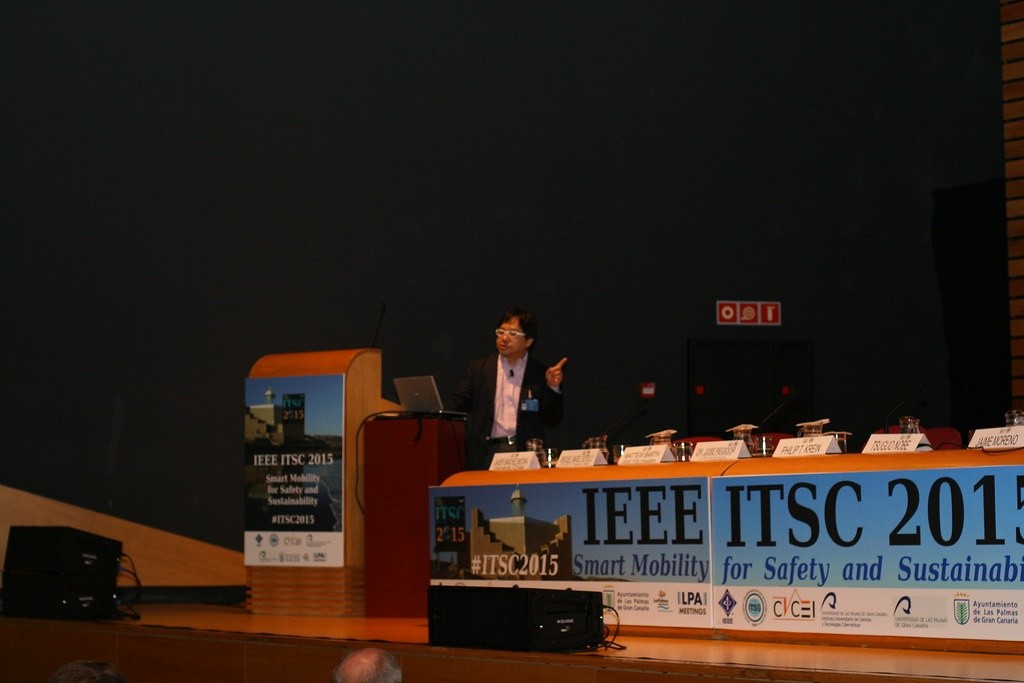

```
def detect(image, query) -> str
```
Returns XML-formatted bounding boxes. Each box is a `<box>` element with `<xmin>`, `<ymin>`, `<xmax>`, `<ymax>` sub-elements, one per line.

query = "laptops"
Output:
<box><xmin>394</xmin><ymin>375</ymin><xmax>468</xmax><ymax>415</ymax></box>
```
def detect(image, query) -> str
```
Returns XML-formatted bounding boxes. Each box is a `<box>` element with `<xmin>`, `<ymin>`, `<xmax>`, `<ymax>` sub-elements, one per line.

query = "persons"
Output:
<box><xmin>440</xmin><ymin>307</ymin><xmax>568</xmax><ymax>468</ymax></box>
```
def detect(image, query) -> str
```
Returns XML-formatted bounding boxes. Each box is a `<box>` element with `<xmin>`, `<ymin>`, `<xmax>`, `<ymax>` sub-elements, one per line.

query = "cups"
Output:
<box><xmin>899</xmin><ymin>416</ymin><xmax>920</xmax><ymax>433</ymax></box>
<box><xmin>1004</xmin><ymin>410</ymin><xmax>1024</xmax><ymax>426</ymax></box>
<box><xmin>612</xmin><ymin>444</ymin><xmax>629</xmax><ymax>462</ymax></box>
<box><xmin>755</xmin><ymin>436</ymin><xmax>773</xmax><ymax>457</ymax></box>
<box><xmin>547</xmin><ymin>448</ymin><xmax>559</xmax><ymax>468</ymax></box>
<box><xmin>829</xmin><ymin>434</ymin><xmax>846</xmax><ymax>453</ymax></box>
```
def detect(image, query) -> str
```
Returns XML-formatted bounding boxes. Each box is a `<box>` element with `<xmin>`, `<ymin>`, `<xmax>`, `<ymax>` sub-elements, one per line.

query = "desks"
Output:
<box><xmin>439</xmin><ymin>445</ymin><xmax>1024</xmax><ymax>654</ymax></box>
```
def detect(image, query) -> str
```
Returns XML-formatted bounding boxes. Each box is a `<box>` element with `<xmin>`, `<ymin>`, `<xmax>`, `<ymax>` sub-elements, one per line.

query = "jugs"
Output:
<box><xmin>797</xmin><ymin>421</ymin><xmax>822</xmax><ymax>437</ymax></box>
<box><xmin>528</xmin><ymin>438</ymin><xmax>547</xmax><ymax>467</ymax></box>
<box><xmin>582</xmin><ymin>435</ymin><xmax>608</xmax><ymax>463</ymax></box>
<box><xmin>733</xmin><ymin>428</ymin><xmax>754</xmax><ymax>455</ymax></box>
<box><xmin>674</xmin><ymin>442</ymin><xmax>692</xmax><ymax>462</ymax></box>
<box><xmin>649</xmin><ymin>433</ymin><xmax>674</xmax><ymax>454</ymax></box>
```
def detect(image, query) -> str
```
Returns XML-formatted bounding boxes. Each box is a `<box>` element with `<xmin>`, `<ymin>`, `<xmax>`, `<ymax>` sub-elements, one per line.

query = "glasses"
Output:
<box><xmin>495</xmin><ymin>329</ymin><xmax>528</xmax><ymax>338</ymax></box>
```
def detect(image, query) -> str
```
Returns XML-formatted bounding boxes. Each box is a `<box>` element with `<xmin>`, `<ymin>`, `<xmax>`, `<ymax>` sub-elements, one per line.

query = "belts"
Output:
<box><xmin>490</xmin><ymin>435</ymin><xmax>516</xmax><ymax>446</ymax></box>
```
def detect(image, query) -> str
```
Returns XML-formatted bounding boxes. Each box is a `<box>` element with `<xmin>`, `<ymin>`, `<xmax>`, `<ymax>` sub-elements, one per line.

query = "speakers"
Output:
<box><xmin>427</xmin><ymin>585</ymin><xmax>604</xmax><ymax>653</ymax></box>
<box><xmin>0</xmin><ymin>526</ymin><xmax>124</xmax><ymax>622</ymax></box>
